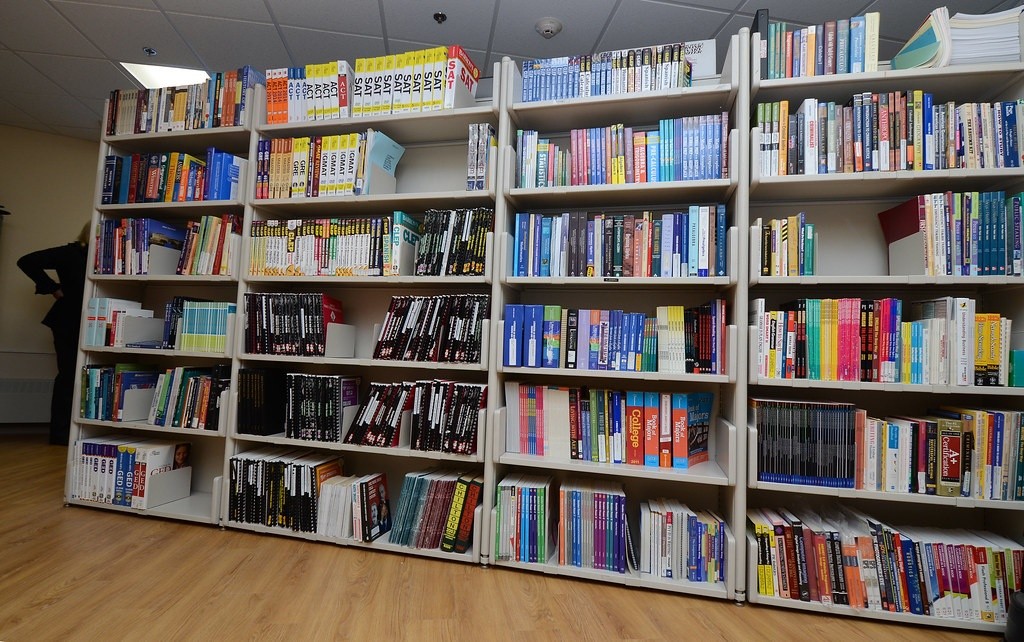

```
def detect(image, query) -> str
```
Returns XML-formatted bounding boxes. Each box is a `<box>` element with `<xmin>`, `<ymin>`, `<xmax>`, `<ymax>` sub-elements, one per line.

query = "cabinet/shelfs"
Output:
<box><xmin>61</xmin><ymin>9</ymin><xmax>1023</xmax><ymax>635</ymax></box>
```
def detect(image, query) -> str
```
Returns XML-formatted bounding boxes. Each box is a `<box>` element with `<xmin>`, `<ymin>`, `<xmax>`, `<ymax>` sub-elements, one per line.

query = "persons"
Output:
<box><xmin>16</xmin><ymin>220</ymin><xmax>93</xmax><ymax>444</ymax></box>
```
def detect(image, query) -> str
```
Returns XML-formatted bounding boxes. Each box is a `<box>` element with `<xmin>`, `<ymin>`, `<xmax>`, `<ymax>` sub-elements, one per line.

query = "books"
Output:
<box><xmin>70</xmin><ymin>6</ymin><xmax>1024</xmax><ymax>624</ymax></box>
